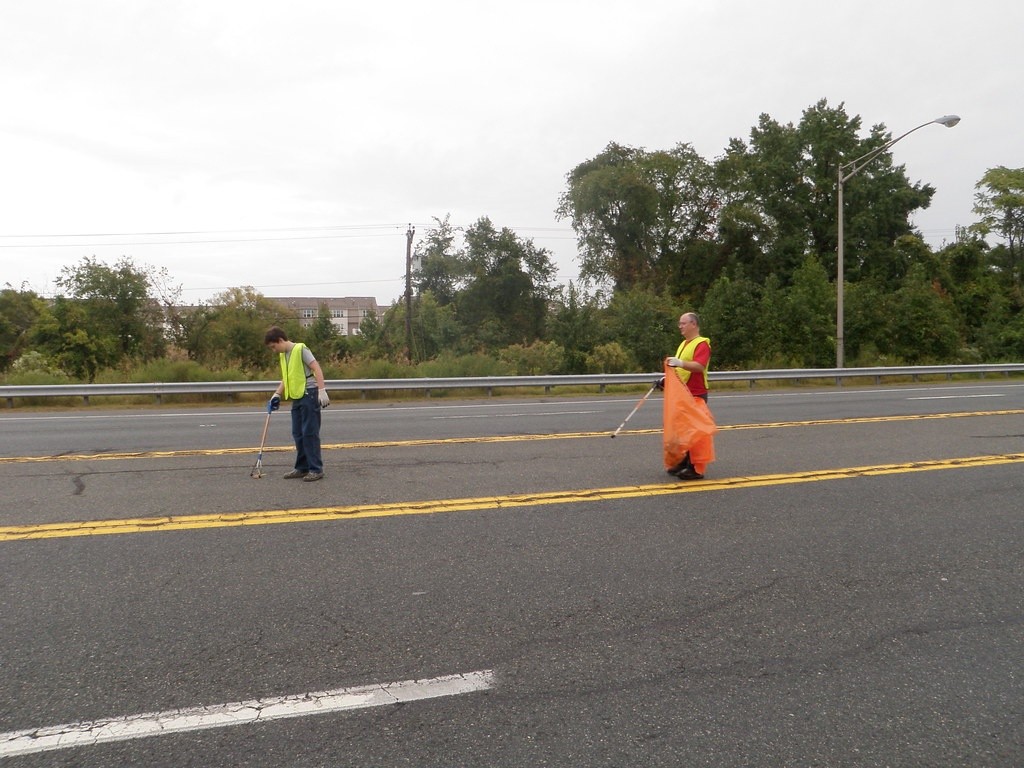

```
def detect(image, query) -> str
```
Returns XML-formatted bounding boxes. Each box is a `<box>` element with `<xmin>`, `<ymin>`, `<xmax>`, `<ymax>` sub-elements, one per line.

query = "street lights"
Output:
<box><xmin>834</xmin><ymin>114</ymin><xmax>963</xmax><ymax>388</ymax></box>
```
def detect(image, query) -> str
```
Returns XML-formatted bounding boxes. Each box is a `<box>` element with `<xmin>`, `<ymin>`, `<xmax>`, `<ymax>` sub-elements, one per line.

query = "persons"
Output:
<box><xmin>653</xmin><ymin>312</ymin><xmax>712</xmax><ymax>479</ymax></box>
<box><xmin>266</xmin><ymin>328</ymin><xmax>330</xmax><ymax>480</ymax></box>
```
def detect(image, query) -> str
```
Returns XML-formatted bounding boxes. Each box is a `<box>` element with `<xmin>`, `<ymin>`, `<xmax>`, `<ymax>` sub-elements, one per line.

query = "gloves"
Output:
<box><xmin>667</xmin><ymin>356</ymin><xmax>682</xmax><ymax>369</ymax></box>
<box><xmin>270</xmin><ymin>393</ymin><xmax>282</xmax><ymax>411</ymax></box>
<box><xmin>318</xmin><ymin>388</ymin><xmax>331</xmax><ymax>408</ymax></box>
<box><xmin>660</xmin><ymin>377</ymin><xmax>665</xmax><ymax>381</ymax></box>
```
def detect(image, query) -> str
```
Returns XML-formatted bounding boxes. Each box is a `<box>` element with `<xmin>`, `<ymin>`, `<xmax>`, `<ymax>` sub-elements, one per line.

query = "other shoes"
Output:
<box><xmin>666</xmin><ymin>463</ymin><xmax>687</xmax><ymax>475</ymax></box>
<box><xmin>283</xmin><ymin>469</ymin><xmax>309</xmax><ymax>479</ymax></box>
<box><xmin>676</xmin><ymin>468</ymin><xmax>705</xmax><ymax>479</ymax></box>
<box><xmin>302</xmin><ymin>470</ymin><xmax>325</xmax><ymax>482</ymax></box>
